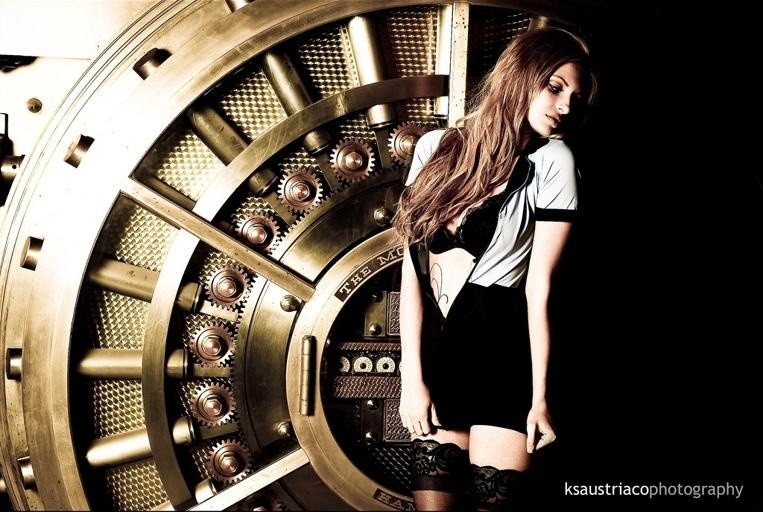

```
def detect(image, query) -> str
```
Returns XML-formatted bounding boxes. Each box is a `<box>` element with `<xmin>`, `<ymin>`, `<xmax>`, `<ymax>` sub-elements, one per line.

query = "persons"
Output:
<box><xmin>388</xmin><ymin>28</ymin><xmax>599</xmax><ymax>512</ymax></box>
